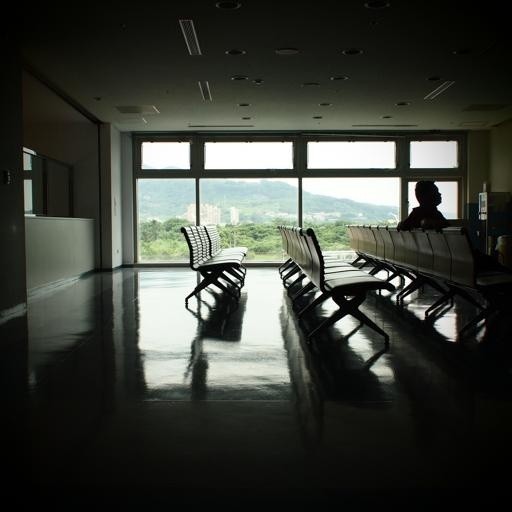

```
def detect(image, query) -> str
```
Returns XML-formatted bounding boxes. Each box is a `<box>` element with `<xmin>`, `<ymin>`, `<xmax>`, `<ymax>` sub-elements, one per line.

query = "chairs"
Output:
<box><xmin>277</xmin><ymin>225</ymin><xmax>511</xmax><ymax>347</ymax></box>
<box><xmin>178</xmin><ymin>225</ymin><xmax>249</xmax><ymax>311</ymax></box>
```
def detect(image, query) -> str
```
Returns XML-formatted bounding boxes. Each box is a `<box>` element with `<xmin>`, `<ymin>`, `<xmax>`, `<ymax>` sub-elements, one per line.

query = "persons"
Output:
<box><xmin>397</xmin><ymin>180</ymin><xmax>504</xmax><ymax>269</ymax></box>
